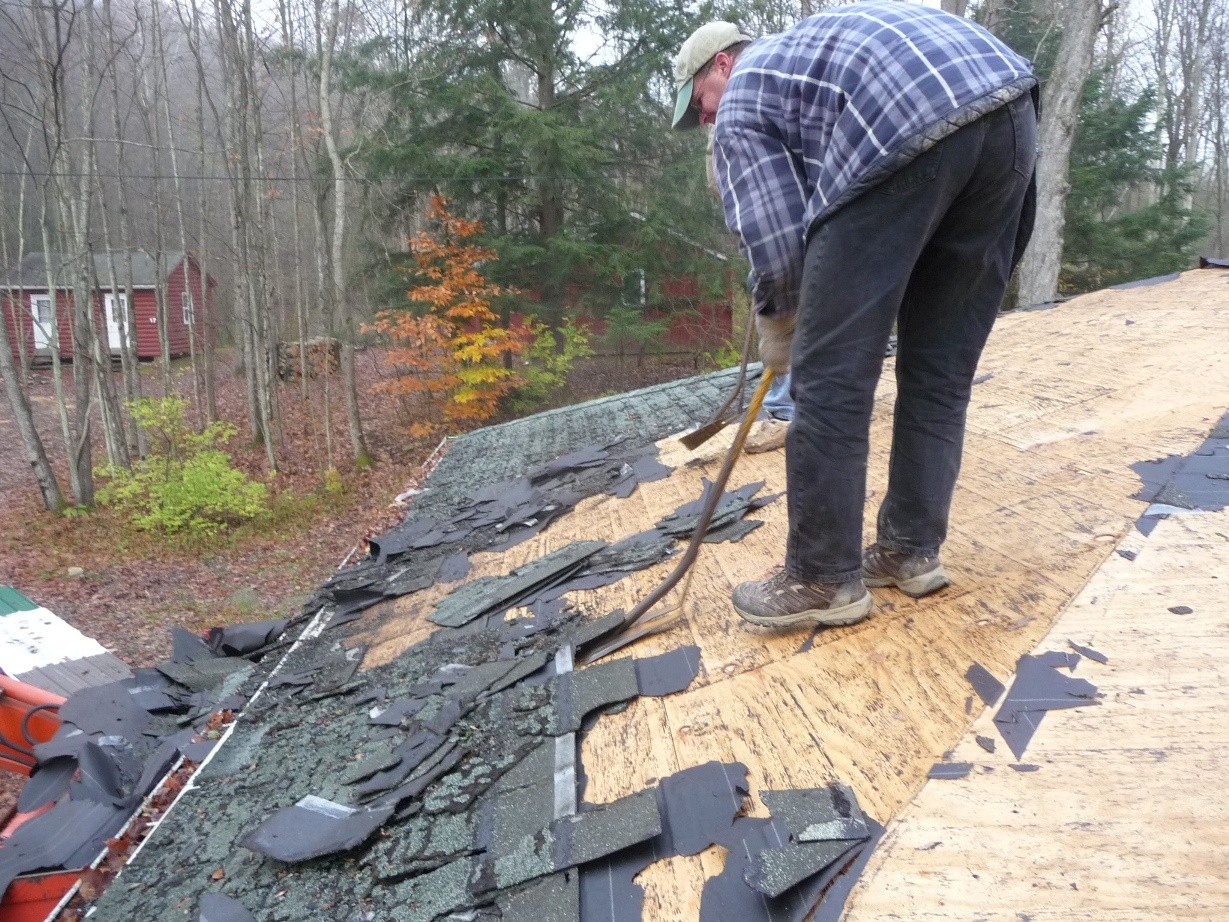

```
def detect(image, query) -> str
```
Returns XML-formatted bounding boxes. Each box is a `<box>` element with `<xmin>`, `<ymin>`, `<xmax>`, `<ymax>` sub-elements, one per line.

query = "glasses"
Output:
<box><xmin>691</xmin><ymin>51</ymin><xmax>730</xmax><ymax>113</ymax></box>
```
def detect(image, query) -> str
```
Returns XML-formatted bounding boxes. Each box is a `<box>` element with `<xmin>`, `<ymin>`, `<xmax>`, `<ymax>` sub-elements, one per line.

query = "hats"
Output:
<box><xmin>671</xmin><ymin>21</ymin><xmax>752</xmax><ymax>130</ymax></box>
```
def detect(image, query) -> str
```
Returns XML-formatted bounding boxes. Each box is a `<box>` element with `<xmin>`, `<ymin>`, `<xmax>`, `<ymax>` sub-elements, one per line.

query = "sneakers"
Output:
<box><xmin>744</xmin><ymin>411</ymin><xmax>792</xmax><ymax>454</ymax></box>
<box><xmin>860</xmin><ymin>543</ymin><xmax>950</xmax><ymax>598</ymax></box>
<box><xmin>732</xmin><ymin>569</ymin><xmax>873</xmax><ymax>625</ymax></box>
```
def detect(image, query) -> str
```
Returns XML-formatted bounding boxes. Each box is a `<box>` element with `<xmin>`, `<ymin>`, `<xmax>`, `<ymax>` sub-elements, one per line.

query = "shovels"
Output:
<box><xmin>579</xmin><ymin>370</ymin><xmax>774</xmax><ymax>656</ymax></box>
<box><xmin>679</xmin><ymin>293</ymin><xmax>755</xmax><ymax>449</ymax></box>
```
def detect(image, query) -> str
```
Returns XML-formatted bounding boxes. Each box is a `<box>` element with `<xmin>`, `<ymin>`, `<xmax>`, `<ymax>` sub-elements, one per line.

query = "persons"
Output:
<box><xmin>671</xmin><ymin>1</ymin><xmax>1043</xmax><ymax>629</ymax></box>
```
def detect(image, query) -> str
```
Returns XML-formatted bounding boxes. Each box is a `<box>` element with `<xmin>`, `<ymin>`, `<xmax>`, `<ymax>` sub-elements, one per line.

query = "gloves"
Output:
<box><xmin>755</xmin><ymin>314</ymin><xmax>798</xmax><ymax>375</ymax></box>
